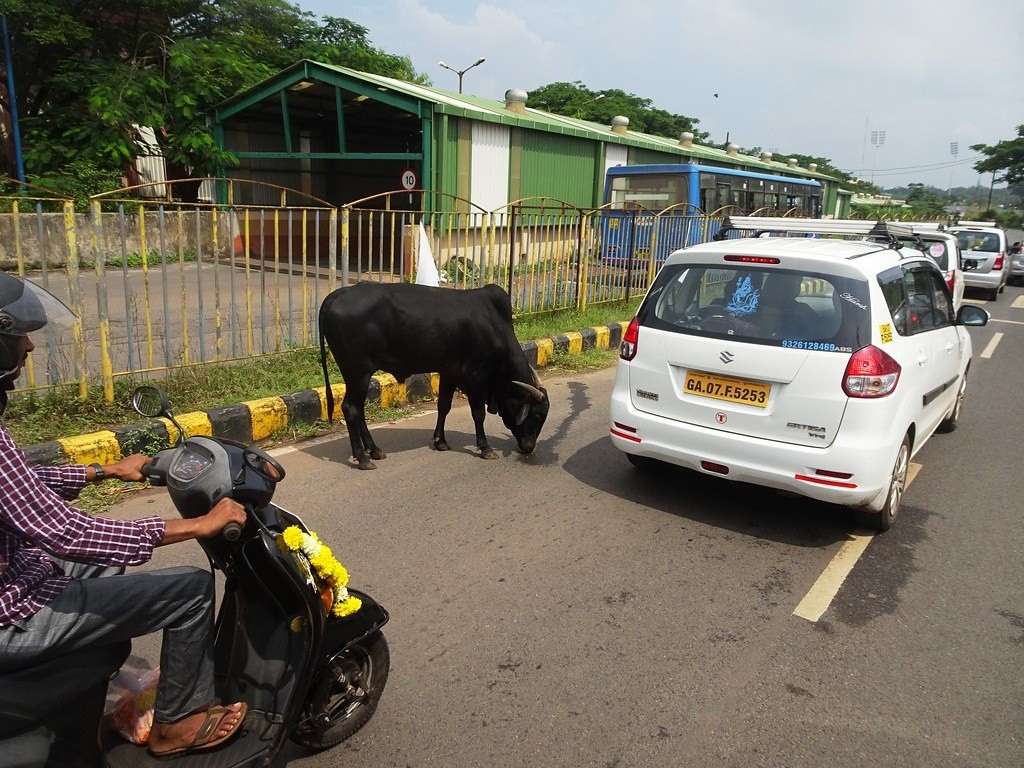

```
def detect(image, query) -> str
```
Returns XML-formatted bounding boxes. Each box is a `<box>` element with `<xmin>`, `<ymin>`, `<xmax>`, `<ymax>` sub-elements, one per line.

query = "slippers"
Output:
<box><xmin>154</xmin><ymin>703</ymin><xmax>247</xmax><ymax>761</ymax></box>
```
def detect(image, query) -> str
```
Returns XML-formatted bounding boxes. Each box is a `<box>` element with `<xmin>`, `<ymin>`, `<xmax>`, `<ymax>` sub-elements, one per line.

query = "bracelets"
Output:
<box><xmin>88</xmin><ymin>462</ymin><xmax>104</xmax><ymax>483</ymax></box>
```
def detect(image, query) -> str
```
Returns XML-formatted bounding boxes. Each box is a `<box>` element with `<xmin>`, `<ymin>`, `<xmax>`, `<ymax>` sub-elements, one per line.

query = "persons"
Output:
<box><xmin>0</xmin><ymin>272</ymin><xmax>248</xmax><ymax>756</ymax></box>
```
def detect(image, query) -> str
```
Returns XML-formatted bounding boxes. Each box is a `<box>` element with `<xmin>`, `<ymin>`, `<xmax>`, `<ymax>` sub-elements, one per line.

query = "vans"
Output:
<box><xmin>856</xmin><ymin>218</ymin><xmax>1024</xmax><ymax>323</ymax></box>
<box><xmin>605</xmin><ymin>215</ymin><xmax>992</xmax><ymax>534</ymax></box>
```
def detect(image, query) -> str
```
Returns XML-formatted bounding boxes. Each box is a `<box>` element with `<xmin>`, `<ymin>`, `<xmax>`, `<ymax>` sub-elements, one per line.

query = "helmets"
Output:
<box><xmin>0</xmin><ymin>272</ymin><xmax>47</xmax><ymax>336</ymax></box>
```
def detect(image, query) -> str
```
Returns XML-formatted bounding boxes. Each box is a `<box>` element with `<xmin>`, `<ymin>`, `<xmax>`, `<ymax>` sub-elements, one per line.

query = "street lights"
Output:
<box><xmin>438</xmin><ymin>57</ymin><xmax>486</xmax><ymax>94</ymax></box>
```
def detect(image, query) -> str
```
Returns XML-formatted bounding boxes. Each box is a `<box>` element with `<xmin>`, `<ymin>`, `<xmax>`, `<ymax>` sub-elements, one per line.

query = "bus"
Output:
<box><xmin>593</xmin><ymin>163</ymin><xmax>824</xmax><ymax>274</ymax></box>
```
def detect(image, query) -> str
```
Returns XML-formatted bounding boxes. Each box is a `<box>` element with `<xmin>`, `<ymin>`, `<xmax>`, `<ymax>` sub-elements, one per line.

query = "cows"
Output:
<box><xmin>317</xmin><ymin>283</ymin><xmax>550</xmax><ymax>471</ymax></box>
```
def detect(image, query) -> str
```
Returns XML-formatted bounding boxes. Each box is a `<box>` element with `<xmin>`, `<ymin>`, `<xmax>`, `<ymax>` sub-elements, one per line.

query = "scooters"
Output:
<box><xmin>0</xmin><ymin>384</ymin><xmax>392</xmax><ymax>768</ymax></box>
